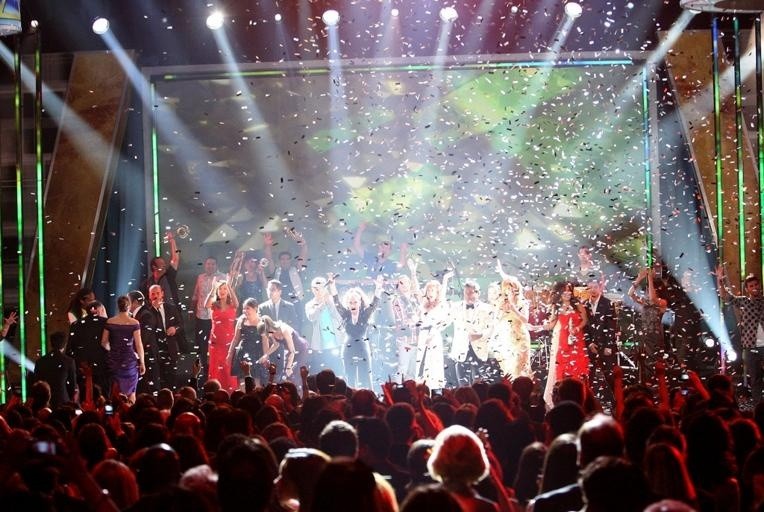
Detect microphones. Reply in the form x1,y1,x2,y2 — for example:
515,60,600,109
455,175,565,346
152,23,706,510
324,272,340,288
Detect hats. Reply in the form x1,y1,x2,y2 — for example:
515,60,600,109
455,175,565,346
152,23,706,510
309,276,326,288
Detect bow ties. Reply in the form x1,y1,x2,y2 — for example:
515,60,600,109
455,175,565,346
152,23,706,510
465,303,475,309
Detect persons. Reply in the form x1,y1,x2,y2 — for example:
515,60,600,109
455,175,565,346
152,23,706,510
578,276,619,399
627,267,668,374
493,258,550,345
0,358,764,512
146,284,184,388
66,302,110,400
0,310,19,342
256,314,309,389
406,258,454,395
256,279,297,334
100,296,146,404
522,281,589,409
713,263,764,407
231,251,268,317
484,281,502,305
263,228,308,340
352,223,408,280
125,290,161,392
32,330,79,407
203,272,239,394
492,277,532,380
195,260,228,379
304,277,346,376
325,272,385,392
226,297,268,388
391,275,421,383
450,281,497,388
67,288,107,324
573,245,605,293
140,231,191,354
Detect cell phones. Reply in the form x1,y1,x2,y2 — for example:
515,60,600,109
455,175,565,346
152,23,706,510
104,401,114,415
28,441,55,456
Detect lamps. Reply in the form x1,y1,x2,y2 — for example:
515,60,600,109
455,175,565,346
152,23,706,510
90,14,109,36
321,9,340,27
563,0,582,18
204,14,223,30
439,7,458,25
